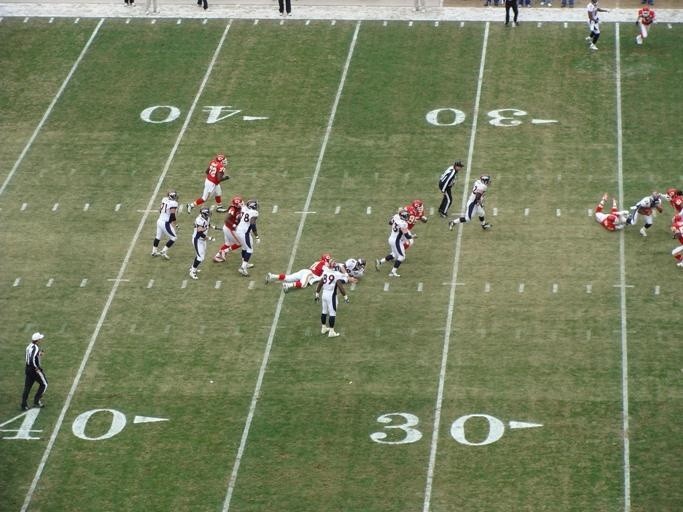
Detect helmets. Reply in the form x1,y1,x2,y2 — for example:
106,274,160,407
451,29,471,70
399,200,423,221
481,176,490,183
232,197,257,209
607,215,618,225
168,193,177,200
321,253,336,268
217,155,227,165
200,208,209,218
651,188,677,203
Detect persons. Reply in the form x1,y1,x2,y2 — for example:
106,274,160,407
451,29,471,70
150,191,178,260
197,0,208,9
438,160,465,218
447,175,494,232
124,0,136,7
189,207,222,280
279,1,292,16
593,186,683,269
21,331,48,411
235,199,261,277
212,197,244,263
186,154,230,215
484,0,656,52
264,199,428,338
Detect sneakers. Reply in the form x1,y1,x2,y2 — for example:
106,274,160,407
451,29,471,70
213,250,226,262
321,327,339,337
603,193,617,207
639,228,647,237
152,250,170,260
238,264,253,275
189,269,201,279
187,204,192,214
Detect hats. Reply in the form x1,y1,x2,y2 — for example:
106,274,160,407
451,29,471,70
455,162,464,166
32,333,43,340
